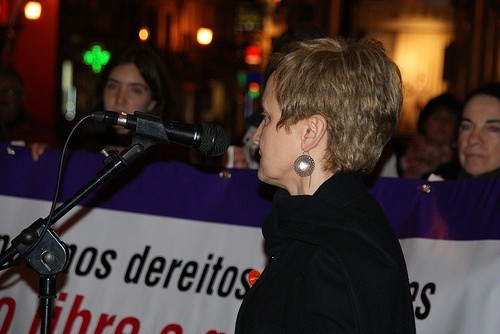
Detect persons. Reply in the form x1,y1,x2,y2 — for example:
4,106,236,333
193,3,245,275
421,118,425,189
0,42,500,180
234,36,416,334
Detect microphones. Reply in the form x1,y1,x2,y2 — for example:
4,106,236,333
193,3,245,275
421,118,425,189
90,111,230,158
